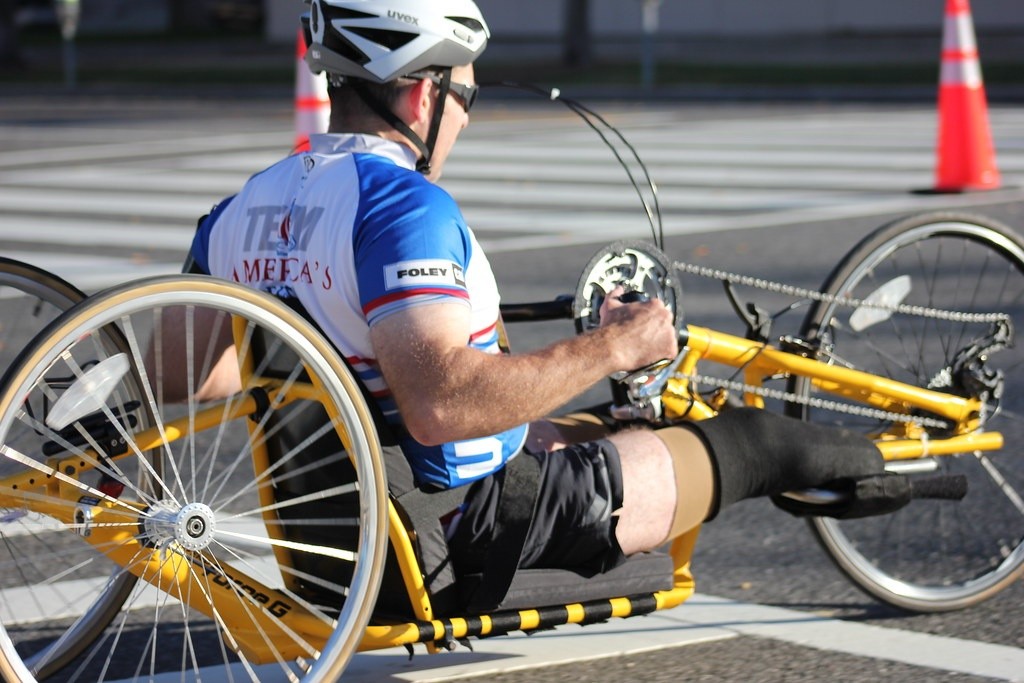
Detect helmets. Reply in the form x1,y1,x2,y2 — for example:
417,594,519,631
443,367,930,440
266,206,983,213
299,0,491,83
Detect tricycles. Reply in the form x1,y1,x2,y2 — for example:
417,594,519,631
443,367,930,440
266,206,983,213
0,74,1023,683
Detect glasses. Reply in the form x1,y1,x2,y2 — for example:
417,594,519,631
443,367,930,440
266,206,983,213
403,73,480,113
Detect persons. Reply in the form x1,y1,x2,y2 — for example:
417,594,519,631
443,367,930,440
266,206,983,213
144,0,885,576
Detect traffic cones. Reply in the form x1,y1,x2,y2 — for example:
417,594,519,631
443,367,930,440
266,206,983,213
911,1,1019,198
288,22,336,160
